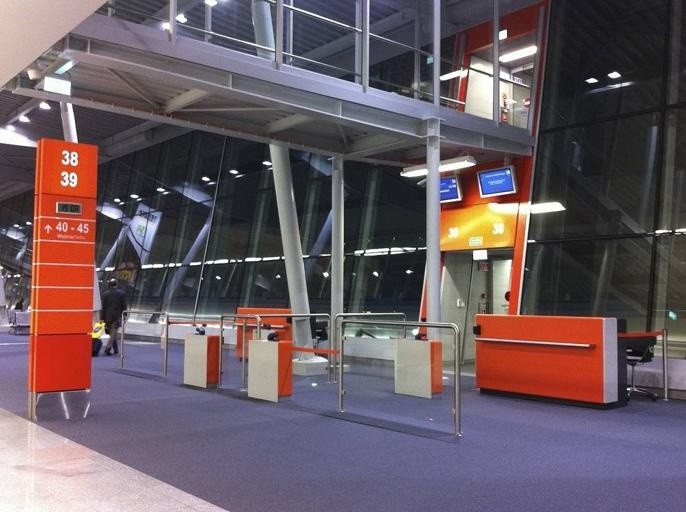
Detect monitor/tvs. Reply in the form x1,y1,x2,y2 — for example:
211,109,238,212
476,165,518,199
440,174,463,203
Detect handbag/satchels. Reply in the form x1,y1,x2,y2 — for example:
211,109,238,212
90,322,106,339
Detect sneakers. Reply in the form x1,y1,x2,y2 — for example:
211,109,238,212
105,350,118,355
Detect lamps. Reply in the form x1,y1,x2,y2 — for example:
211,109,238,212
400,151,477,179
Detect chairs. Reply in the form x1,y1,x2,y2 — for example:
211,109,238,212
6,309,30,335
624,333,658,401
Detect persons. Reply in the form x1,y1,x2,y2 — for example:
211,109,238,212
97,278,127,355
14,297,24,310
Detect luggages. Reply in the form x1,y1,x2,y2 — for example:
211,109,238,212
92,338,102,357
